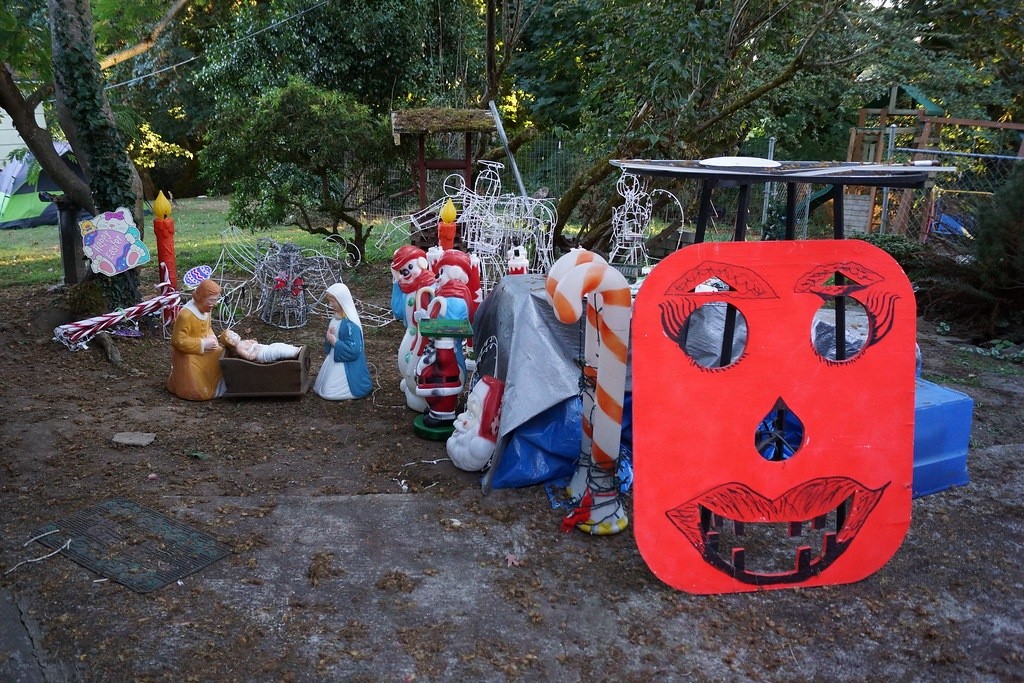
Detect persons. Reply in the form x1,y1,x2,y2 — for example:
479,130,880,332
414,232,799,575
221,329,303,364
167,279,227,400
312,283,372,400
